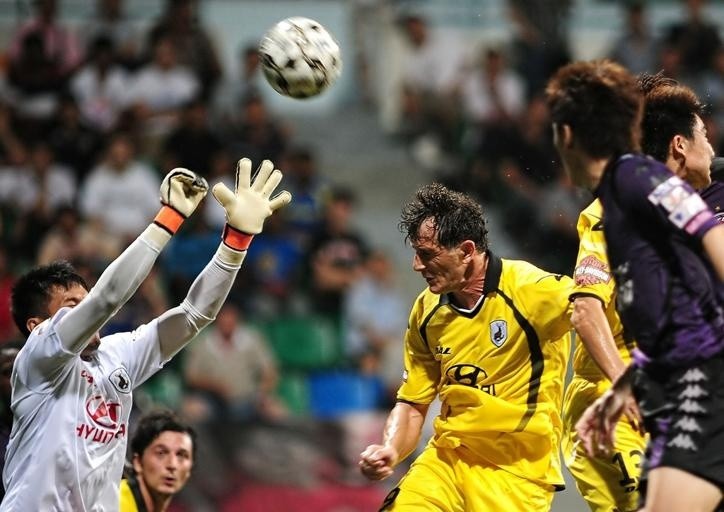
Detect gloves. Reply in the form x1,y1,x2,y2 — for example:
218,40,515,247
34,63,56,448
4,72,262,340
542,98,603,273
153,167,210,236
211,157,293,251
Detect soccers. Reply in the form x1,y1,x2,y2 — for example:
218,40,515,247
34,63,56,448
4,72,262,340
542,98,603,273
258,17,343,98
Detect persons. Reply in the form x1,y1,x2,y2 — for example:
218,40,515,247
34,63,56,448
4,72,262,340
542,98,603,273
1,1,723,512
